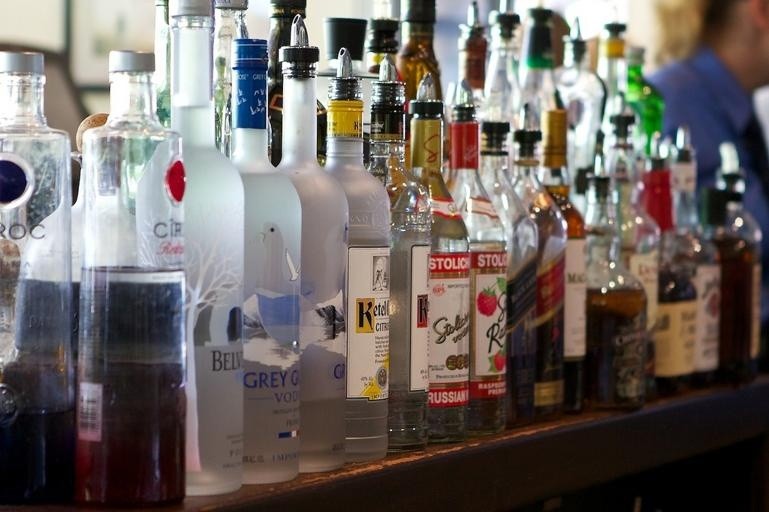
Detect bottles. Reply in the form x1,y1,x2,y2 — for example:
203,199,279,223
442,105,508,438
265,0,327,169
276,14,348,473
672,148,722,385
326,17,372,170
406,102,471,445
538,110,583,414
562,18,606,217
222,41,302,483
524,8,565,127
457,1,486,108
583,176,651,412
718,170,762,382
169,1,244,494
516,129,568,416
325,49,392,465
0,53,73,511
486,0,530,127
152,2,170,132
603,23,632,168
640,156,696,392
481,123,538,430
368,0,399,86
369,80,433,454
611,117,659,402
211,1,251,160
399,1,444,106
77,51,183,511
624,47,663,154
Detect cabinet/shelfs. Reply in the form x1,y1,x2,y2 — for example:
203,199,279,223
0,373,769,512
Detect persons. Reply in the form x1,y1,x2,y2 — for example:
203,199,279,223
647,0,769,324
